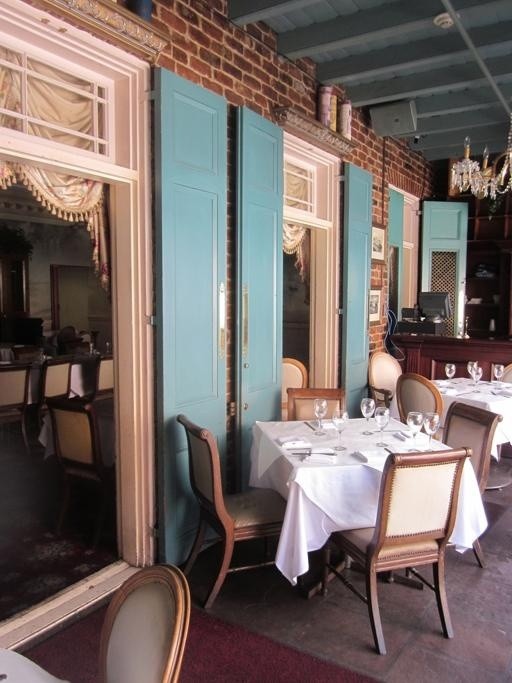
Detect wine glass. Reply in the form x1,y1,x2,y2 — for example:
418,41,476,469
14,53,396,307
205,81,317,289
331,408,350,452
423,413,442,453
359,398,375,436
470,366,483,394
405,411,424,453
374,407,392,448
445,364,457,389
493,364,505,390
466,361,478,386
312,398,328,437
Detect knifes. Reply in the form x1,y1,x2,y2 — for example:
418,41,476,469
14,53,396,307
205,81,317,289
292,451,337,456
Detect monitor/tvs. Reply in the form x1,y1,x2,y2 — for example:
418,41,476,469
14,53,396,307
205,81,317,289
418,290,453,321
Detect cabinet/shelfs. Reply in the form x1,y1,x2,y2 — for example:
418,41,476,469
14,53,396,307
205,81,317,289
460,196,512,337
385,333,512,380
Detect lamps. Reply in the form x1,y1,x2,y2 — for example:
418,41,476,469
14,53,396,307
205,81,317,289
450,104,511,198
433,12,457,30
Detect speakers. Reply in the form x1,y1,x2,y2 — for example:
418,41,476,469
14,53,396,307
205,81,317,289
369,99,418,137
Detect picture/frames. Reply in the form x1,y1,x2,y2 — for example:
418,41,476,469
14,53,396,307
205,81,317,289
50,262,112,351
368,285,383,325
371,220,389,266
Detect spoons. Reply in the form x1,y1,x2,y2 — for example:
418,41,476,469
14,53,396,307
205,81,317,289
490,390,501,395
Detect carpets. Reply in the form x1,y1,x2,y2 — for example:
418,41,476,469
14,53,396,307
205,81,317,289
22,596,388,682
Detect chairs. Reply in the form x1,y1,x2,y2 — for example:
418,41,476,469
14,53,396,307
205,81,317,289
321,446,474,654
397,372,444,440
44,401,116,548
11,346,46,358
368,350,403,407
388,400,505,578
177,414,305,608
0,363,33,451
497,363,512,382
286,387,347,420
73,356,113,412
281,357,309,421
17,357,72,444
51,325,91,352
99,562,192,682
